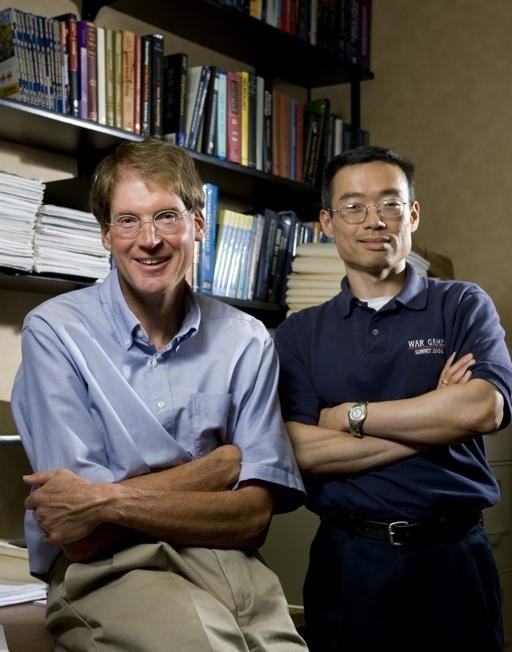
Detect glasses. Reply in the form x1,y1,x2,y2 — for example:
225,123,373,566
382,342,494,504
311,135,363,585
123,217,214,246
326,199,408,222
105,207,191,241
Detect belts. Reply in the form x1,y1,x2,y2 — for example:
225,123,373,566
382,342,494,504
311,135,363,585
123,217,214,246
336,513,482,545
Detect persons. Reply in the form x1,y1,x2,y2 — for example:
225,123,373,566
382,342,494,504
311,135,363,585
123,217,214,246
9,142,314,652
265,146,511,651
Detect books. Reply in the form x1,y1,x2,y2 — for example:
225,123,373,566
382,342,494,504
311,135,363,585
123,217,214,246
1,7,352,184
359,130,370,147
230,1,368,66
0,536,48,609
1,171,430,321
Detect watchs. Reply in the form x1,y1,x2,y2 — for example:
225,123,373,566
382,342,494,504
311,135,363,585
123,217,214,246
346,393,372,439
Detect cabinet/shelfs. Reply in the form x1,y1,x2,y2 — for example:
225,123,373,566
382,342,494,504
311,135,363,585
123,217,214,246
0,0,374,513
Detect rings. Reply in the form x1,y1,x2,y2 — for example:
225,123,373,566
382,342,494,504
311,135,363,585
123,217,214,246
440,378,449,384
